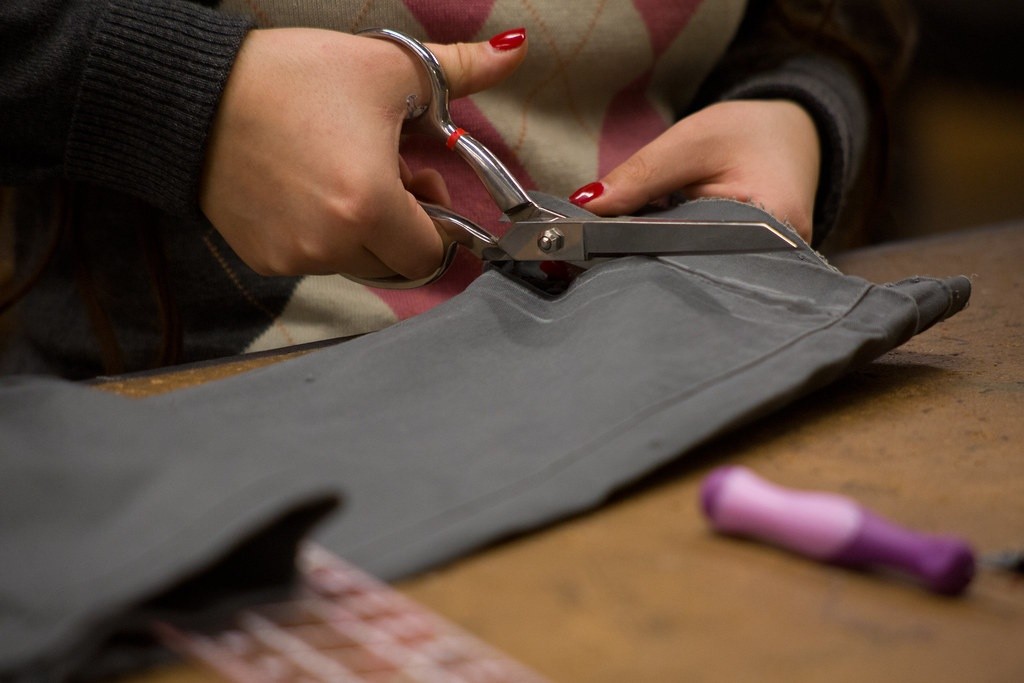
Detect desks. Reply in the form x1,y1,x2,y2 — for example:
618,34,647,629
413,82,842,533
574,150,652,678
0,225,1023,682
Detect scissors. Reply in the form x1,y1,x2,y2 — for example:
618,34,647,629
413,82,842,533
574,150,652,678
336,28,801,292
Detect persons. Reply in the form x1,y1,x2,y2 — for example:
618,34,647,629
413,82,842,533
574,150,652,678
1,1,935,389
